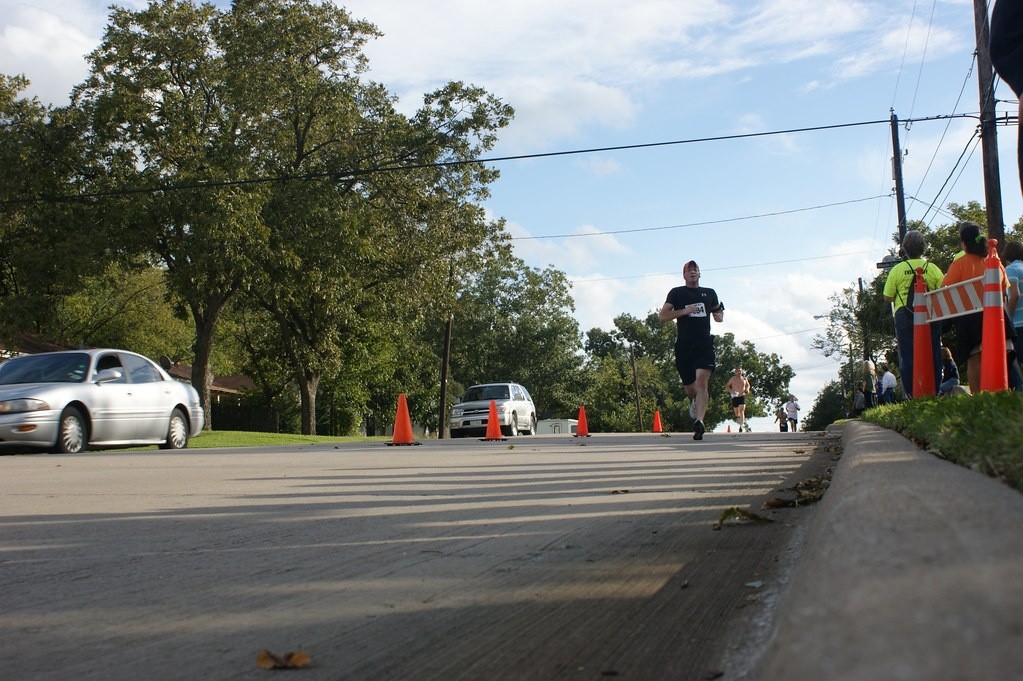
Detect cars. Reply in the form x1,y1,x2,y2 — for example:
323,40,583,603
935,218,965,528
0,348,205,455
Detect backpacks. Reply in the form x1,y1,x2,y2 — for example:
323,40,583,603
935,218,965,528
896,260,929,318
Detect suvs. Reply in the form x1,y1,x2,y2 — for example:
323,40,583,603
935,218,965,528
449,382,538,438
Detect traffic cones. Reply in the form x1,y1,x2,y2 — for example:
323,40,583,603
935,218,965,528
979,239,1008,391
478,400,509,442
651,411,663,432
912,266,936,397
572,405,592,437
727,424,731,432
384,393,422,446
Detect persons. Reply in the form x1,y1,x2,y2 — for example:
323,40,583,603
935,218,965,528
882,229,945,401
726,367,750,433
852,360,898,418
659,260,725,440
785,395,800,433
938,221,1023,395
774,408,788,432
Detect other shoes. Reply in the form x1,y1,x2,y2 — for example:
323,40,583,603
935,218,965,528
689,397,697,419
693,419,705,440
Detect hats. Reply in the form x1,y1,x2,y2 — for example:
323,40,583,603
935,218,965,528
683,260,699,269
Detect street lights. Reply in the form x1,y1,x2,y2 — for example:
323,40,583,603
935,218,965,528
813,315,870,360
